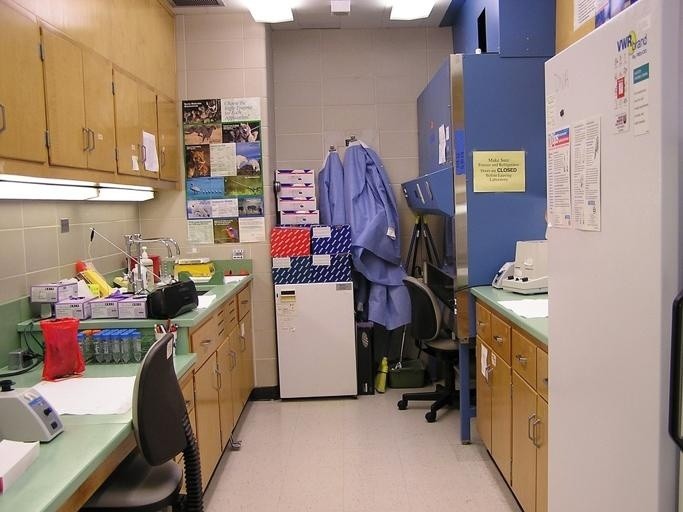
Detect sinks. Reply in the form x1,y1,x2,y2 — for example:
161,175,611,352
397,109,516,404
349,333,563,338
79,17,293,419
195,277,211,298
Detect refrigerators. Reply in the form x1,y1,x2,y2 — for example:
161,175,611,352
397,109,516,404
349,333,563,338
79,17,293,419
544,0,681,512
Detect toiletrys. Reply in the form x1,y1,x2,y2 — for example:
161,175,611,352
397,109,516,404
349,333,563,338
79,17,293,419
141,247,154,289
133,262,148,292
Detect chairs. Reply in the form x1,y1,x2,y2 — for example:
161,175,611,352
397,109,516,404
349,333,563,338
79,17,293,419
396,276,477,422
78,332,205,512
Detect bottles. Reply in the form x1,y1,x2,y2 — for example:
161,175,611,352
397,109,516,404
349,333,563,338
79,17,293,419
74,328,140,364
133,263,148,289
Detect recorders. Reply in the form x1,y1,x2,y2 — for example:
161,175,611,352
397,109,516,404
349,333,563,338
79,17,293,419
145,280,200,320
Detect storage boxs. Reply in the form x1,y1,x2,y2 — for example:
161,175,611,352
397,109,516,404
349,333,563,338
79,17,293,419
268,166,354,289
389,357,425,390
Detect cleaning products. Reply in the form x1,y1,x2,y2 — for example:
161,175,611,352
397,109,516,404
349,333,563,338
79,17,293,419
374,356,387,393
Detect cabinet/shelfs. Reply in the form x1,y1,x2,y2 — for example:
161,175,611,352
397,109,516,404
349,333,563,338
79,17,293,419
158,94,181,182
510,329,548,511
18,282,257,501
41,21,115,174
0,0,46,167
473,299,513,493
113,63,157,181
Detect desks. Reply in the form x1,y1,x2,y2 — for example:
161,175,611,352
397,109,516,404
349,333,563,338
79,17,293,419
1,351,197,511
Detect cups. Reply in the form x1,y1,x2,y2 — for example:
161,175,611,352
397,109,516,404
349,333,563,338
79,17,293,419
154,331,177,358
38,318,85,381
158,258,175,282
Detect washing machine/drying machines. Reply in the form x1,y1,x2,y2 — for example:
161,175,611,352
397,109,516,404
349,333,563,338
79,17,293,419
275,282,359,400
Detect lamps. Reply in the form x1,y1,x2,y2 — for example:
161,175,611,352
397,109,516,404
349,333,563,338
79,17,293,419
0,177,155,203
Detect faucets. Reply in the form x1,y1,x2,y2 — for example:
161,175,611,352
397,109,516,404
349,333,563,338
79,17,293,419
141,237,181,257
133,239,172,259
